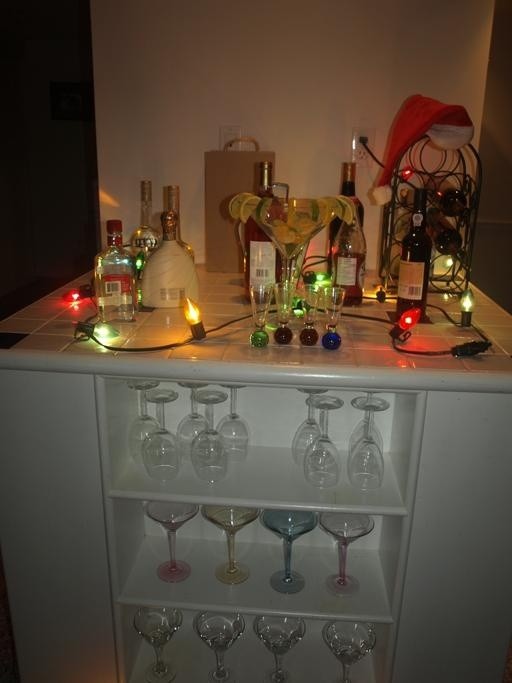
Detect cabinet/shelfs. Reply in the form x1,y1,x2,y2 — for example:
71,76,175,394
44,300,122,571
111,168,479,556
0,370,510,679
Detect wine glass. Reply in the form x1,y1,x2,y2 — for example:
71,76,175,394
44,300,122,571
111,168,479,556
290,387,393,496
143,501,199,583
319,620,377,683
253,614,308,683
192,610,247,682
200,504,261,586
258,509,317,595
125,379,251,490
132,605,184,683
247,281,347,350
319,511,376,599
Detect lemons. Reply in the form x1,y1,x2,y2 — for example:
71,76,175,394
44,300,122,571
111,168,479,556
227,192,356,258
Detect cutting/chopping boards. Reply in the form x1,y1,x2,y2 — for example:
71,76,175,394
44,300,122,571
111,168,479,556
204,136,278,274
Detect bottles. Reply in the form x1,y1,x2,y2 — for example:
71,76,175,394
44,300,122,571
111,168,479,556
395,186,432,323
89,178,199,323
243,160,282,304
326,160,367,307
